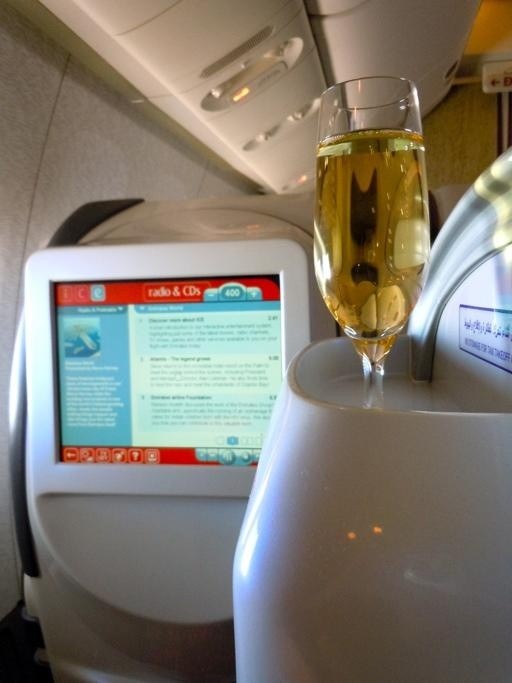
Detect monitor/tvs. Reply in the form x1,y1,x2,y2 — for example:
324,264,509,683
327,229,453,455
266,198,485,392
22,239,310,497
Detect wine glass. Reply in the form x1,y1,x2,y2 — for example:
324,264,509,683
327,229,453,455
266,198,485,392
311,72,434,400
